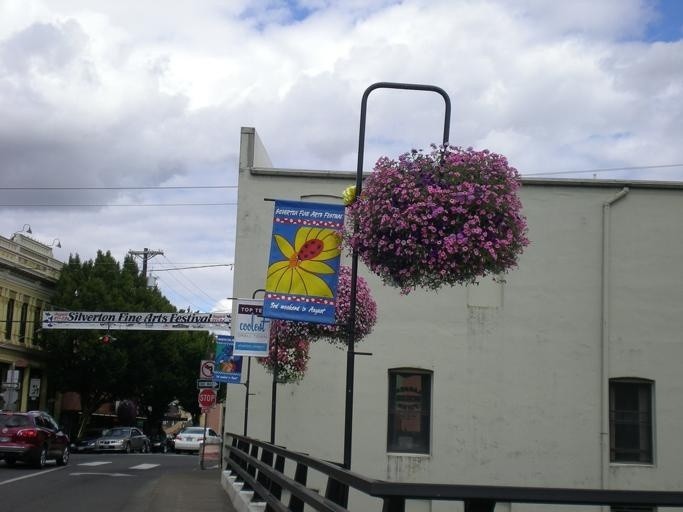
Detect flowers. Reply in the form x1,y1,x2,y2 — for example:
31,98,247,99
253,318,312,388
331,140,530,298
278,264,378,347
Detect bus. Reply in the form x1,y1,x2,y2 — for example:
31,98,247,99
155,411,192,443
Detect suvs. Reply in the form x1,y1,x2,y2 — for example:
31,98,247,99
0,409,70,469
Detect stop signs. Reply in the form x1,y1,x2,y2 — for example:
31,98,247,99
199,391,217,409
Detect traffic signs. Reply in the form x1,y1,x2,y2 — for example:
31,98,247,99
198,380,220,389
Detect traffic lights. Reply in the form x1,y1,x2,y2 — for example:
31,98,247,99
99,335,115,343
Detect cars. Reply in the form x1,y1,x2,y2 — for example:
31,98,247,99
174,427,217,454
72,427,167,454
